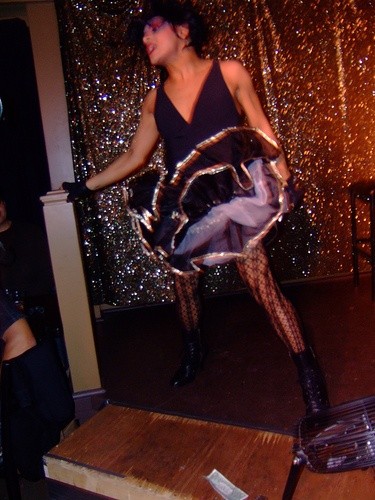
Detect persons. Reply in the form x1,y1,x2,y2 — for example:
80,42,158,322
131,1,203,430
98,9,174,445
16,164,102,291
0,188,76,500
61,0,334,431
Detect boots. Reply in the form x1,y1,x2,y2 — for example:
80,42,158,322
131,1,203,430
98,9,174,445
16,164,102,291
293,347,331,436
171,329,212,386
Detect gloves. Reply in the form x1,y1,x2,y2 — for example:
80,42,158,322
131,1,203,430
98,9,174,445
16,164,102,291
61,180,94,202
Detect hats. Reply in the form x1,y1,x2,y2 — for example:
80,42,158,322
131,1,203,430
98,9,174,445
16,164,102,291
127,0,209,50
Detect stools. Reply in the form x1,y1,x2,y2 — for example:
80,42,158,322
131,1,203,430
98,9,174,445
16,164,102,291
350,179,375,287
